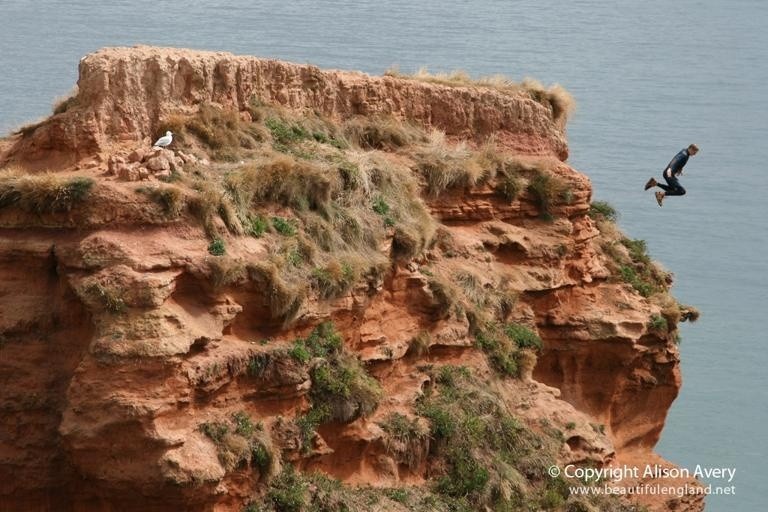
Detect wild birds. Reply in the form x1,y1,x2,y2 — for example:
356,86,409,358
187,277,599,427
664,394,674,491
154,130,175,148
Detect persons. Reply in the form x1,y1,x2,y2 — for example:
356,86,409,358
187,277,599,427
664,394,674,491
644,143,698,207
